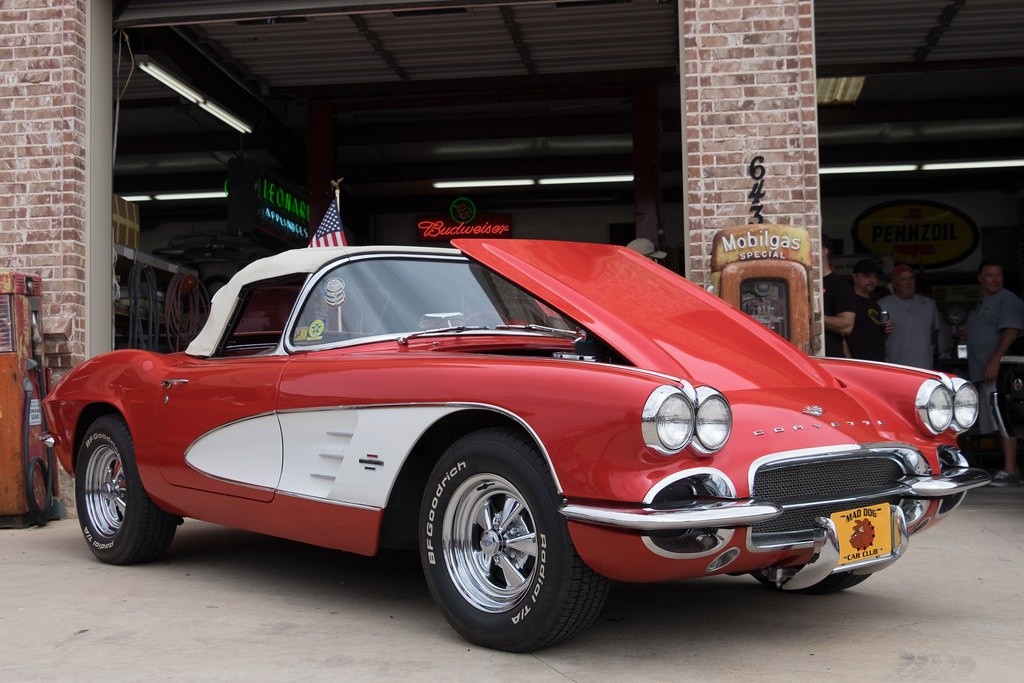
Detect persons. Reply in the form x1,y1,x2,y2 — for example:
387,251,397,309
627,238,656,262
957,262,1024,487
817,235,939,370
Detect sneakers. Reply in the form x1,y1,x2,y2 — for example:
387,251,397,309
987,470,1024,487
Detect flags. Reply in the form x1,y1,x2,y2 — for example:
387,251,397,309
308,199,347,246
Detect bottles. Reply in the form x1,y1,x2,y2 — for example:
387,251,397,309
958,330,966,345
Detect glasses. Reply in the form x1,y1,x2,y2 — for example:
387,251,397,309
645,244,658,256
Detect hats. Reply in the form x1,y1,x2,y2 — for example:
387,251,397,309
854,259,885,276
626,239,668,259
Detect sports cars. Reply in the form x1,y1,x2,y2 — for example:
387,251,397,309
36,235,993,657
